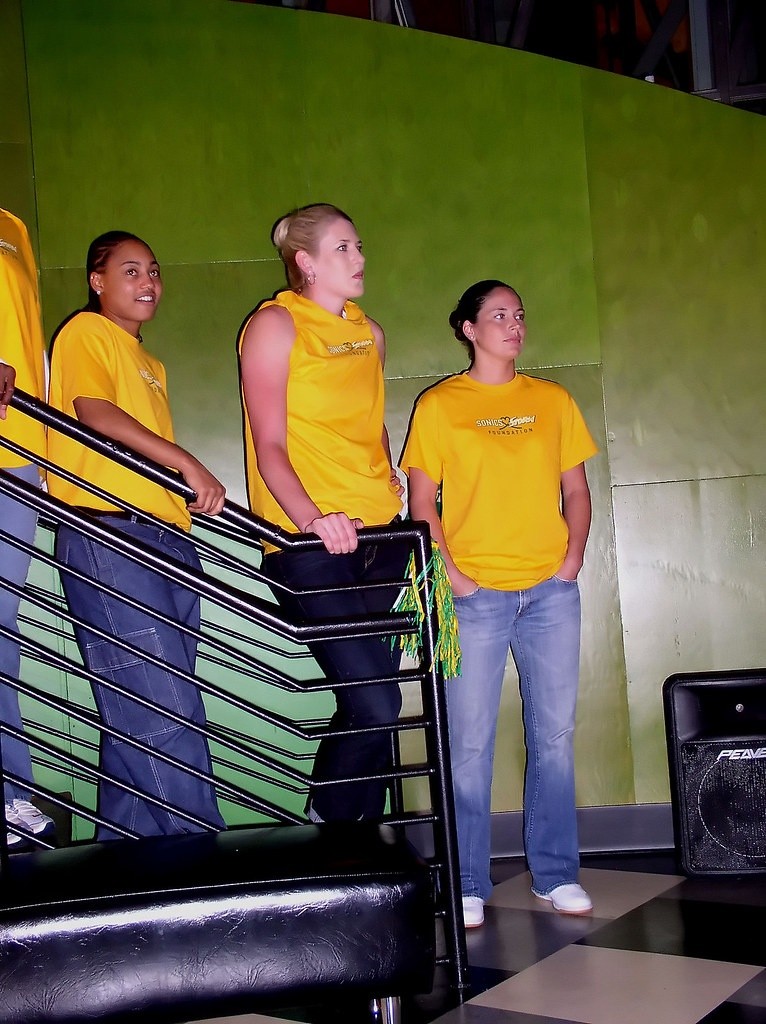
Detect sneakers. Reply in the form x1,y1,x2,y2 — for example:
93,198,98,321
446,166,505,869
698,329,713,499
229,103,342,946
5,805,35,848
533,884,593,915
463,894,485,928
13,799,55,837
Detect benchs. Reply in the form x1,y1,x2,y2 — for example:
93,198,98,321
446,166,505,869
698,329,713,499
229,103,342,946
0,821,438,1023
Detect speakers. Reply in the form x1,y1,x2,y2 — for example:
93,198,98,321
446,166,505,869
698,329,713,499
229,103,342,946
661,668,766,884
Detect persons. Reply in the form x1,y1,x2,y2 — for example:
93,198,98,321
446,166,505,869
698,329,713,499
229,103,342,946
46,230,227,841
237,204,409,823
0,207,55,846
399,281,599,928
